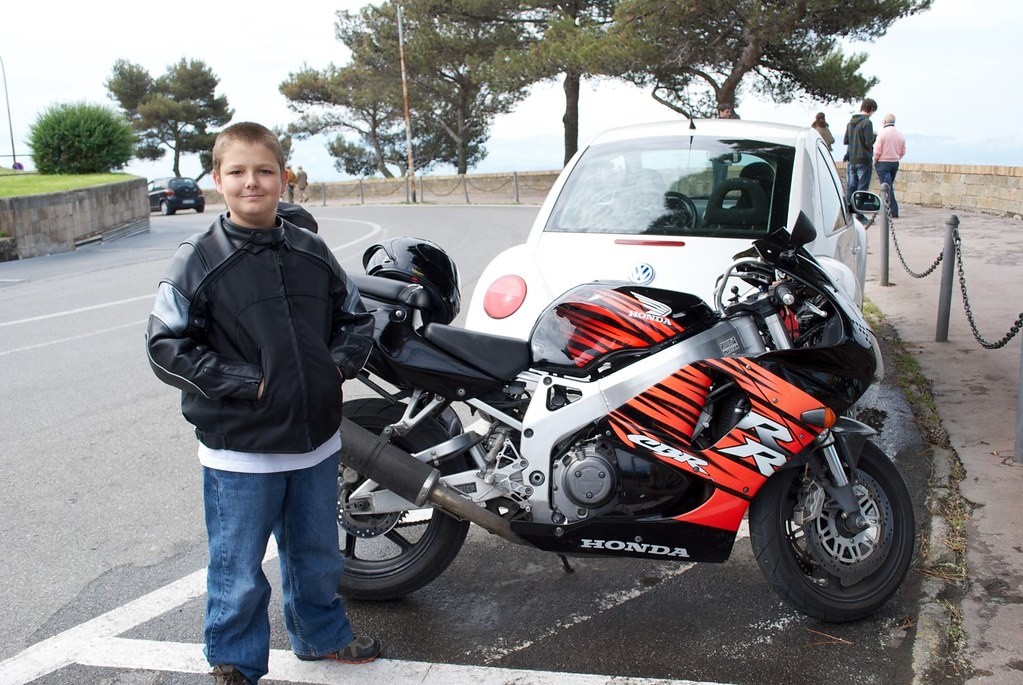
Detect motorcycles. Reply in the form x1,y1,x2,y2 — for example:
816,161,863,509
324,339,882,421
331,236,916,625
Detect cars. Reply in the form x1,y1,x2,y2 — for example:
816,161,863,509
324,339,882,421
461,116,884,355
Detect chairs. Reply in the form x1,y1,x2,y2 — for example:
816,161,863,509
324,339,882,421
701,178,768,230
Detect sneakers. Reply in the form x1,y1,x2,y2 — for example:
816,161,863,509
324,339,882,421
207,663,258,685
294,633,379,664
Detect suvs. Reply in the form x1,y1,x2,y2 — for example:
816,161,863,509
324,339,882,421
148,178,205,216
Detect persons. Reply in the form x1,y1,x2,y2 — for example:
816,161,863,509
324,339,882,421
717,105,731,118
844,98,878,214
812,112,834,153
284,166,310,204
874,114,906,217
145,123,381,684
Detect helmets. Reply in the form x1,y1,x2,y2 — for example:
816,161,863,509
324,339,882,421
362,236,461,326
